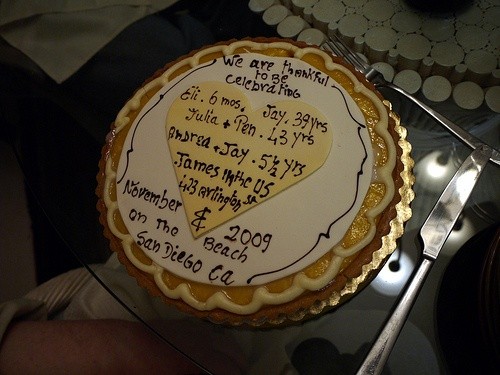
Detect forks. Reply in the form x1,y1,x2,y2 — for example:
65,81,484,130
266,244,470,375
323,33,500,165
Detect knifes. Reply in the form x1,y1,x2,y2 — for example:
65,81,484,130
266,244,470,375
356,144,494,375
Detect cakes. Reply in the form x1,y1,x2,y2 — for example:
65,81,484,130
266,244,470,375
96,39,414,325
